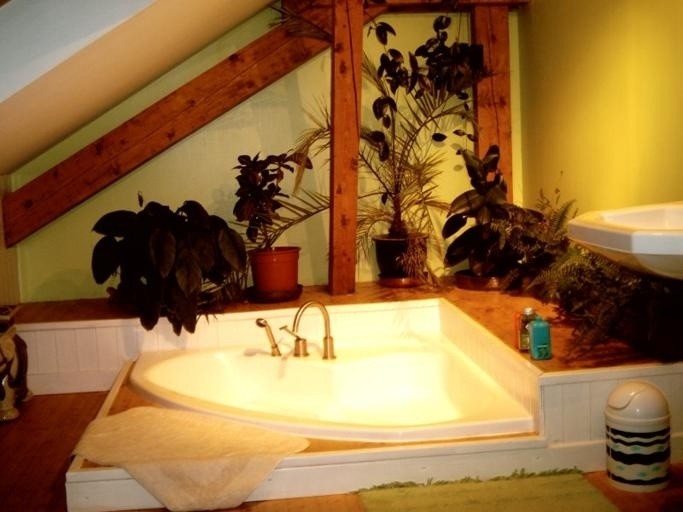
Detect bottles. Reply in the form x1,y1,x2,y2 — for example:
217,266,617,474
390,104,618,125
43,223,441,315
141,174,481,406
527,316,550,360
517,309,538,351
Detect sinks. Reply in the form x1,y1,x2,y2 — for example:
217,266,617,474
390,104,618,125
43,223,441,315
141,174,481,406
130,351,532,436
561,199,682,280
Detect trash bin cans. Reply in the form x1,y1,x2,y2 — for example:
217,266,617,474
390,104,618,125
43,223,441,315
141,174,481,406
603,381,671,495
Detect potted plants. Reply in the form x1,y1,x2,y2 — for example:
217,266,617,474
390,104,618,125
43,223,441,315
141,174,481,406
349,16,494,281
445,134,541,291
231,145,313,300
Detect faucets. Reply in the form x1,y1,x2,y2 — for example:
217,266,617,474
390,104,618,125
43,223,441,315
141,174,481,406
290,299,338,362
256,316,283,361
